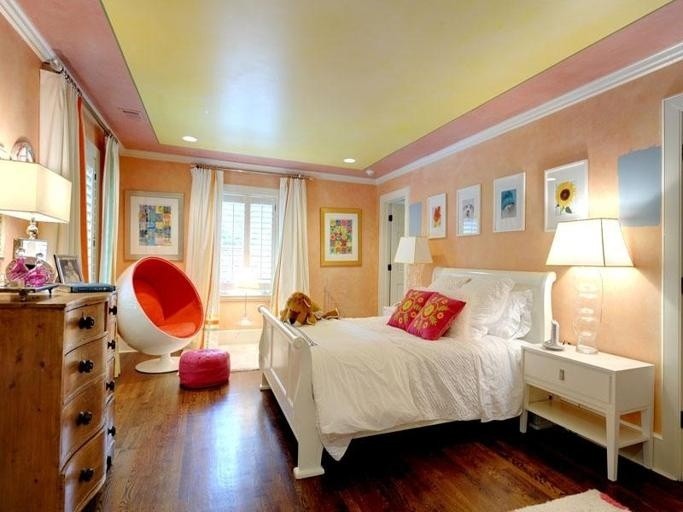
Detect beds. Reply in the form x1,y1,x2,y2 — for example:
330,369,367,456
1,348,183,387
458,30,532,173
257,267,558,481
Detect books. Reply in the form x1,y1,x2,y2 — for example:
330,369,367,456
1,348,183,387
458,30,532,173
70,282,117,293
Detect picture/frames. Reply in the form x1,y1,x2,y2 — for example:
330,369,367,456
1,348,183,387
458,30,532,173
426,192,448,240
492,170,527,234
123,189,185,260
455,183,482,237
319,207,363,267
54,255,84,283
543,158,589,232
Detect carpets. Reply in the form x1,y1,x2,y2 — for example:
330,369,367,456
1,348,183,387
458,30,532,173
508,489,631,511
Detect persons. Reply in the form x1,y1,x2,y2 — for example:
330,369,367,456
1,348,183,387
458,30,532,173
63,262,80,283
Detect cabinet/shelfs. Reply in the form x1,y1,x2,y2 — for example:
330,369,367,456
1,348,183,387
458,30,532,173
1,290,117,512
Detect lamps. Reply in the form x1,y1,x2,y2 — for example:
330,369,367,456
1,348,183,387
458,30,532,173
0,159,73,239
543,219,635,354
394,237,433,288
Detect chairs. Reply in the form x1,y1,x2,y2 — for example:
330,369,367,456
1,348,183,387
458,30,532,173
115,255,204,373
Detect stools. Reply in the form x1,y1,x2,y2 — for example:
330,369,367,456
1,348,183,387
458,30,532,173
178,349,230,390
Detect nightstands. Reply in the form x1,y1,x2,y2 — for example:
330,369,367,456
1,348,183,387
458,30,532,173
519,343,655,481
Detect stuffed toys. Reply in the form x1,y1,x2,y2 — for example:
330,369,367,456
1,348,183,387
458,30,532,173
278,292,320,328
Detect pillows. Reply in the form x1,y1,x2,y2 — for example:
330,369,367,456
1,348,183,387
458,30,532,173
443,279,516,339
488,289,534,339
428,274,472,292
387,289,436,330
406,293,466,339
